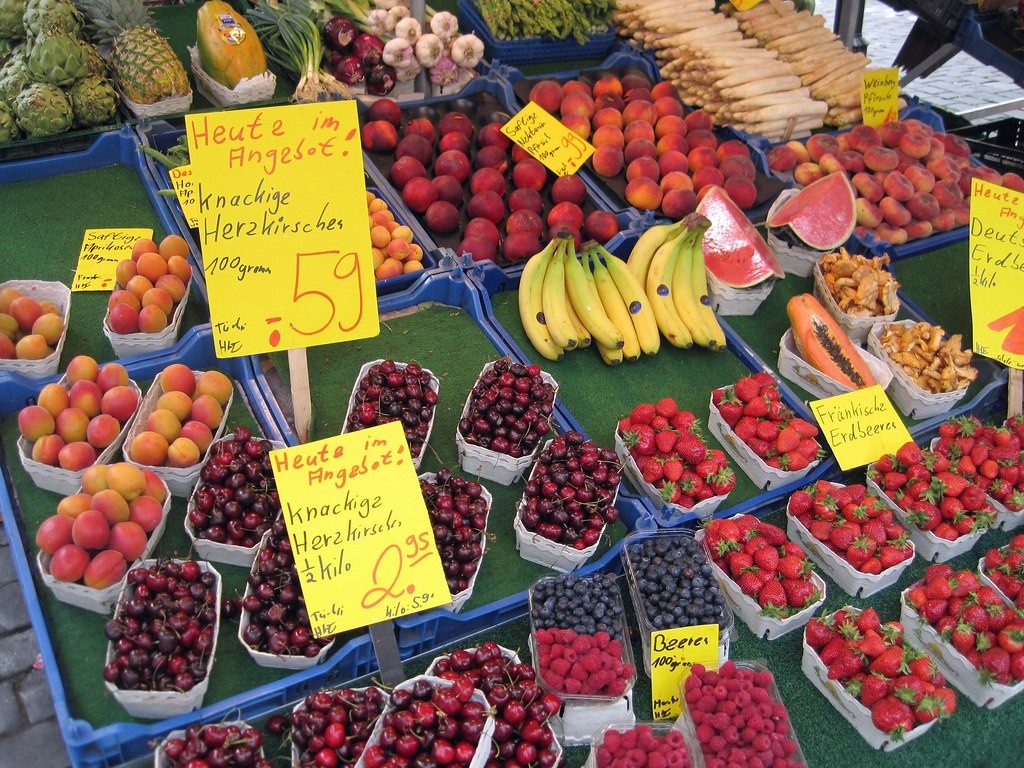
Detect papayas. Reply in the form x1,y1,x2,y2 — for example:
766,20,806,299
785,293,877,391
196,0,267,90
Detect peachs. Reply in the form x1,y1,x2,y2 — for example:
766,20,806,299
531,73,1023,247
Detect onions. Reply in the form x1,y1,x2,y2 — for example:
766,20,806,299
322,17,396,97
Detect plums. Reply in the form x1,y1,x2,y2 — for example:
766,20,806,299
0,234,232,589
366,192,425,280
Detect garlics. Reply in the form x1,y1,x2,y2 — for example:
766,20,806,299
330,0,485,85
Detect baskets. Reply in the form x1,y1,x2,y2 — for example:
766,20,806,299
458,0,616,64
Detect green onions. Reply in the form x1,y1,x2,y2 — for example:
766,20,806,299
241,1,353,103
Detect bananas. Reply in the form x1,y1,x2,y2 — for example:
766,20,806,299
516,214,727,366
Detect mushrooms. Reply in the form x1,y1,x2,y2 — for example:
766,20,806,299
817,246,977,395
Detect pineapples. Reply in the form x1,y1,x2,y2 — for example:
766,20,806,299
76,0,191,105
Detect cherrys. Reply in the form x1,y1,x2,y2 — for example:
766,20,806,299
101,358,621,767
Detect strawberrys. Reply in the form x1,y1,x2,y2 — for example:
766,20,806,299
619,373,1024,742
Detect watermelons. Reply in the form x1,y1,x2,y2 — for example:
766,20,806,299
692,186,784,290
768,171,858,251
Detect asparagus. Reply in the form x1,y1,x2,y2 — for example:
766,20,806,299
475,0,617,46
